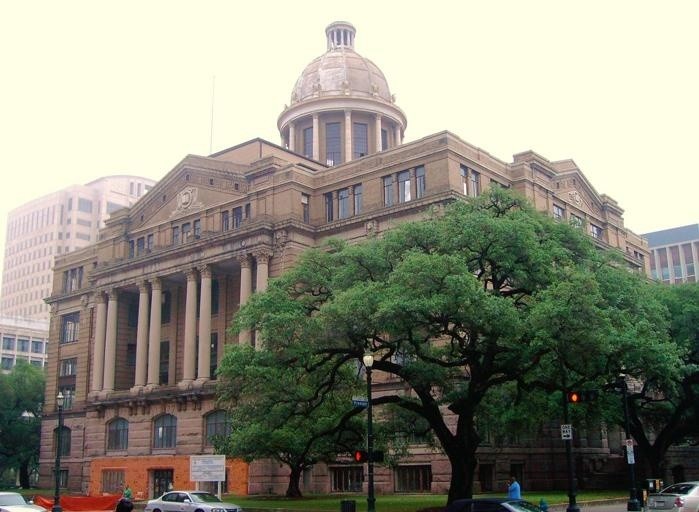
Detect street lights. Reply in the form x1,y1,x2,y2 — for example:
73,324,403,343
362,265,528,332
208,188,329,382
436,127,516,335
51,391,65,512
362,351,376,511
619,361,641,511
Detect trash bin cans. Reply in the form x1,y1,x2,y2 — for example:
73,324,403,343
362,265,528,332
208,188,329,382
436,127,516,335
341,500,356,512
630,489,644,508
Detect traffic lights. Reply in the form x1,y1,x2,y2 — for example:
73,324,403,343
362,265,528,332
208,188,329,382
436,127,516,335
566,390,598,402
355,451,384,463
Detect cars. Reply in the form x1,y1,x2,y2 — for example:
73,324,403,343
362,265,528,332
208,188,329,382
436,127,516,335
0,492,47,512
644,481,699,512
416,498,547,511
145,490,241,512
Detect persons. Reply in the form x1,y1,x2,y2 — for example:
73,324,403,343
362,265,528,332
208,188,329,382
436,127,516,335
115,483,134,512
507,476,522,499
